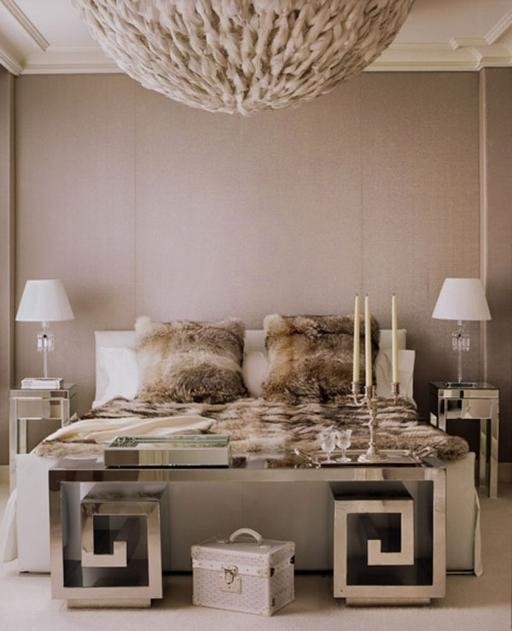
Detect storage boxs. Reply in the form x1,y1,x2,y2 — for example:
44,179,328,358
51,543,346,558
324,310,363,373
191,526,295,617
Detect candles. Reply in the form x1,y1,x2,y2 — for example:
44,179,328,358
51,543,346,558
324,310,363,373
351,294,399,386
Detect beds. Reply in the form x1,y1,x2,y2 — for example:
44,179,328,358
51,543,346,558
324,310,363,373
11,329,482,576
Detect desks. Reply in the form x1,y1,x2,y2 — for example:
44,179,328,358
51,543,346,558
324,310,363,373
44,452,449,610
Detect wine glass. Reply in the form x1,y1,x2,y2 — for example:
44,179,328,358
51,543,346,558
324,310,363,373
335,429,352,461
319,432,337,464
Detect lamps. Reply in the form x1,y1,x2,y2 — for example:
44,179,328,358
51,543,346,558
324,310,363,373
15,279,75,379
431,274,493,386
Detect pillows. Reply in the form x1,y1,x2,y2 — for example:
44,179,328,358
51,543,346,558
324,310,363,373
260,312,383,404
131,312,250,408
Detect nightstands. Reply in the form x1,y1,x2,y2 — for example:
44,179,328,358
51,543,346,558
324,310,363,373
9,384,75,453
430,381,501,501
